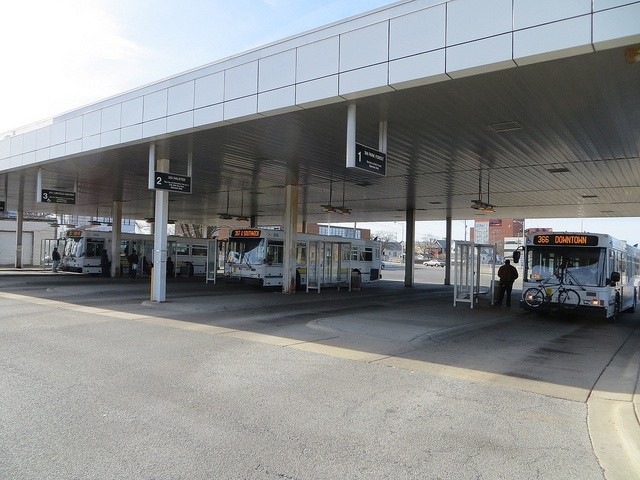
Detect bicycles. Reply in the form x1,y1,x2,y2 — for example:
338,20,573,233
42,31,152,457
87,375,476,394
523,277,581,311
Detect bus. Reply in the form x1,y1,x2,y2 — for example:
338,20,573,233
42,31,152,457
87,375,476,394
222,228,385,292
513,232,640,320
57,228,218,277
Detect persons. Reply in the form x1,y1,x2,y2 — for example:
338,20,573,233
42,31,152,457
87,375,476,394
143,256,148,275
128,250,138,280
166,257,173,277
101,250,111,278
496,260,518,307
52,247,61,273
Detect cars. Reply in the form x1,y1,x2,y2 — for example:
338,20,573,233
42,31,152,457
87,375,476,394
423,259,446,267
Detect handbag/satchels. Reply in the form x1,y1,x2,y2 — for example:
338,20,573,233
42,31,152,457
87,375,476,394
132,264,138,269
